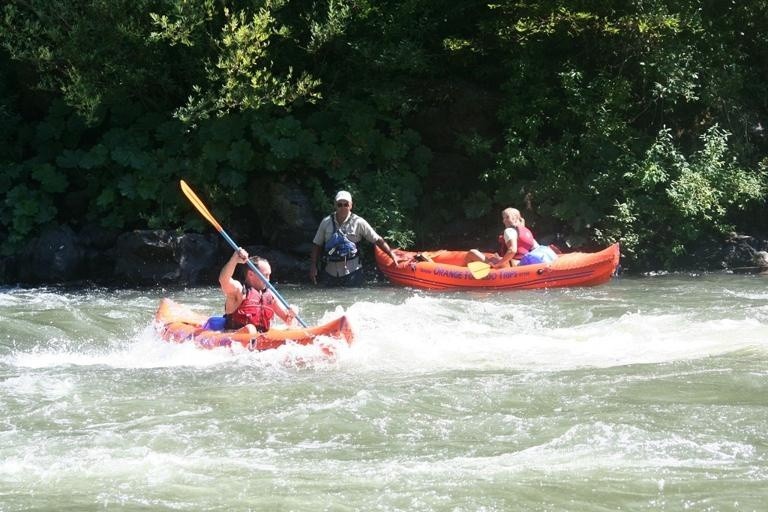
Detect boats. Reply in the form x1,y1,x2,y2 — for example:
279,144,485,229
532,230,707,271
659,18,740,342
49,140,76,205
152,297,353,367
374,241,620,295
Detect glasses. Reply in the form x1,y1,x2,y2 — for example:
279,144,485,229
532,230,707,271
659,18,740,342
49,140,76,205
338,203,350,207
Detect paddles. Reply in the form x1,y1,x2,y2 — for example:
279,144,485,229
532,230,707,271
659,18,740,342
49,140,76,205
467,256,503,280
179,179,309,327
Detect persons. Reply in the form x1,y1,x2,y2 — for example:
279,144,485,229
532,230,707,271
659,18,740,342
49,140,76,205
488,207,540,270
219,246,299,336
310,189,401,290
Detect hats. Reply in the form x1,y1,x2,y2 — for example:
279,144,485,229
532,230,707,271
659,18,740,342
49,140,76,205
335,191,352,202
